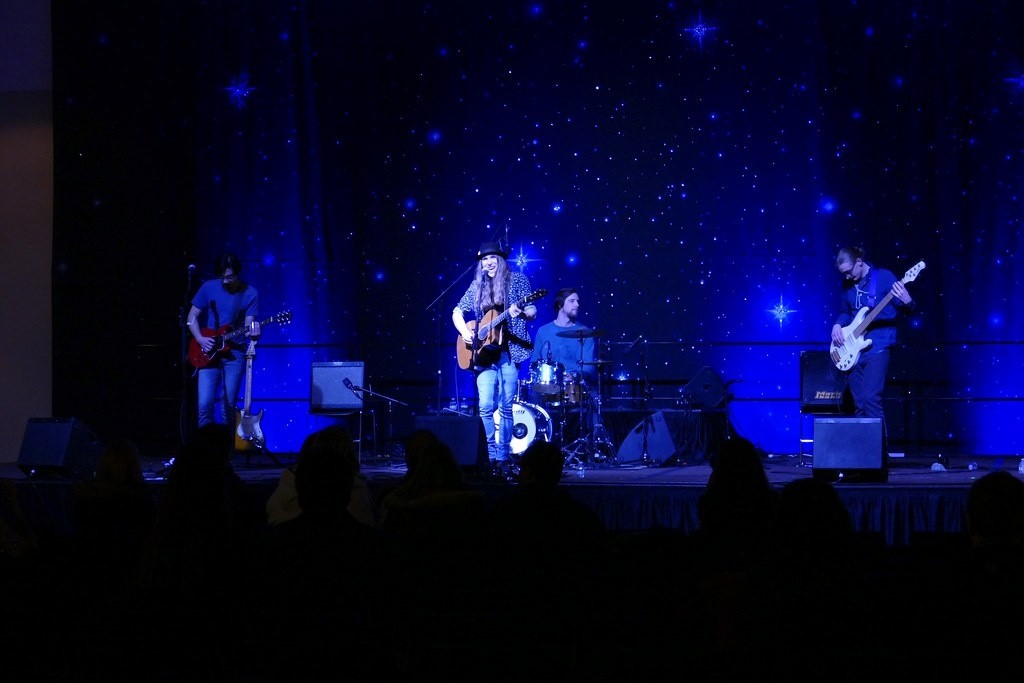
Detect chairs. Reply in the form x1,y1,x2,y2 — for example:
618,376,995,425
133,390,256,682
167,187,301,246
799,350,849,467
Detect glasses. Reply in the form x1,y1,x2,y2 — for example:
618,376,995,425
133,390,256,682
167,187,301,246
845,263,856,274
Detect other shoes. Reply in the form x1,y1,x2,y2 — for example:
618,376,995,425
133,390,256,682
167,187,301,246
491,461,519,476
497,462,515,481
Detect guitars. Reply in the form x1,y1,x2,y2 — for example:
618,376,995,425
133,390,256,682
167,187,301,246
234,337,265,459
188,310,298,368
455,288,549,374
829,260,926,372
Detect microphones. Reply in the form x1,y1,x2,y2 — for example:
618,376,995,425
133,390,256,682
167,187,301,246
343,377,360,398
188,265,195,271
481,267,490,276
547,342,552,360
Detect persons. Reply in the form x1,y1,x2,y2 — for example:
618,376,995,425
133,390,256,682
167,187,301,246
829,245,918,440
530,288,599,443
0,414,1024,683
452,242,538,476
186,252,261,431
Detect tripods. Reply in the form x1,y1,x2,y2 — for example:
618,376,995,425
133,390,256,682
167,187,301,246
560,335,617,468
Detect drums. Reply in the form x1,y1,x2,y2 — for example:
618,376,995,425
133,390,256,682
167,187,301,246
541,370,584,411
493,402,553,457
528,361,562,396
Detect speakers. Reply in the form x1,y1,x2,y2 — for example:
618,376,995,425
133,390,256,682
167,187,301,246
311,362,365,411
16,416,104,481
812,418,887,485
414,416,488,476
617,366,730,465
799,350,854,414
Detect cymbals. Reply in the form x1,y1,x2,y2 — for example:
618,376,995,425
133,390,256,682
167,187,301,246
555,328,610,339
578,360,614,367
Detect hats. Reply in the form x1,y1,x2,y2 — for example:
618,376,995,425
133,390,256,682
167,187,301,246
476,243,507,260
